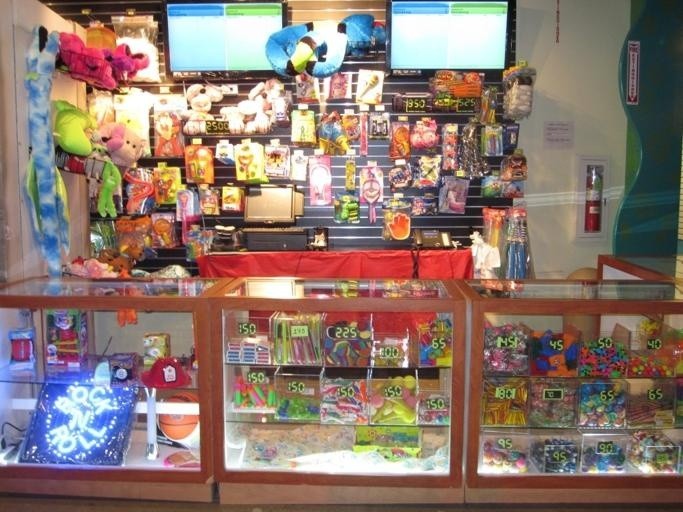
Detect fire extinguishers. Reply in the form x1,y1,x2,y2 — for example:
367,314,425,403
584,166,602,233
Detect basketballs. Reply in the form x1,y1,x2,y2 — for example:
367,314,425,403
157,391,199,440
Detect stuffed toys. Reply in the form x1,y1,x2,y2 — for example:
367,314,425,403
20,20,151,281
264,13,383,77
183,78,289,136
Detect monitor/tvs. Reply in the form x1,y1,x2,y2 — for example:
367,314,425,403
244,182,296,225
384,1,513,80
161,0,288,80
